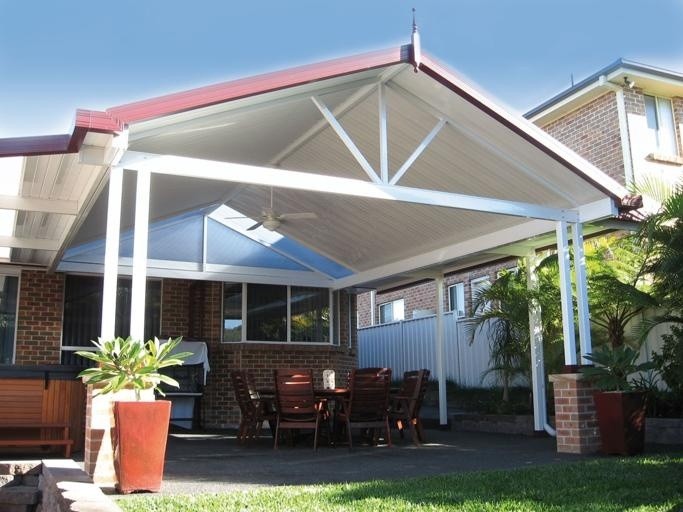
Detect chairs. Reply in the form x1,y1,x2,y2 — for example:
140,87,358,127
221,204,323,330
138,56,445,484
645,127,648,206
230,364,429,454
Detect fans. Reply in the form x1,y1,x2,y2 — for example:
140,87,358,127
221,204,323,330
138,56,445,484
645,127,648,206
221,188,317,232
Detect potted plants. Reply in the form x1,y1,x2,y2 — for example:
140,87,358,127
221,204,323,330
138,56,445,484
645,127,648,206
578,343,651,454
72,333,194,498
